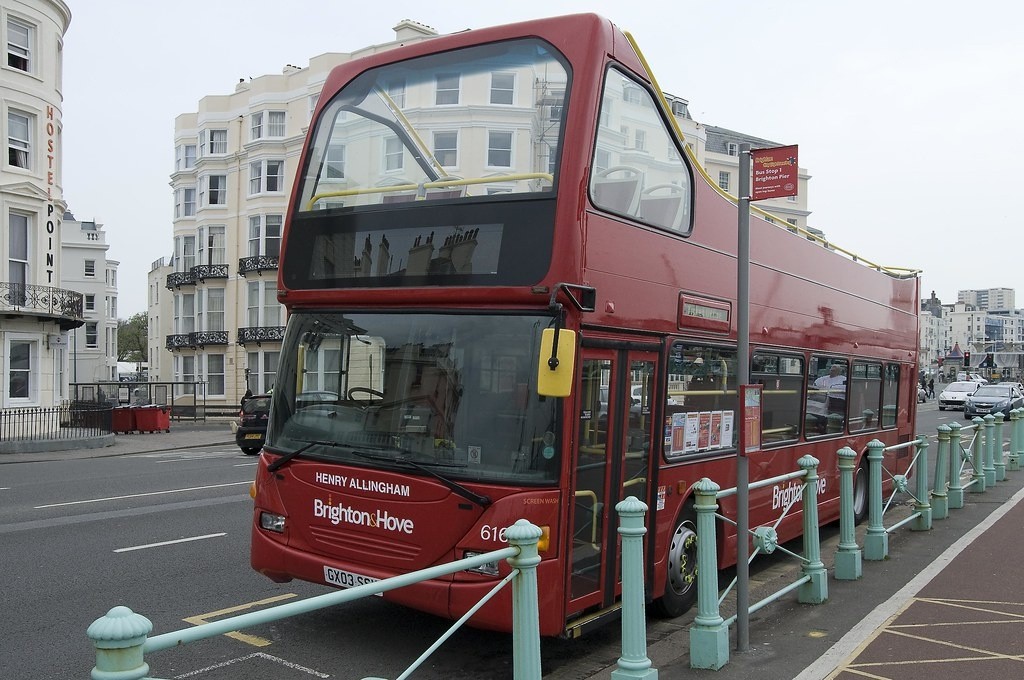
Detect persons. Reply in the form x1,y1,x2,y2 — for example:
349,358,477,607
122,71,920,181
1001,370,1010,382
966,367,970,380
812,364,847,388
942,373,945,379
938,376,940,383
922,376,936,399
987,370,991,383
241,389,252,406
1017,376,1024,388
267,383,274,394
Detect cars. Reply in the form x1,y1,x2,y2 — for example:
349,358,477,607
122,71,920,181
997,382,1024,394
917,387,928,403
939,374,996,411
236,392,344,455
133,385,148,396
964,385,1024,421
599,385,679,423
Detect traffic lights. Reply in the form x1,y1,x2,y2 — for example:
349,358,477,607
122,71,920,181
964,351,970,366
987,354,994,367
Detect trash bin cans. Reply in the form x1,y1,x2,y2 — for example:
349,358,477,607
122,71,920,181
111,406,137,435
134,406,170,435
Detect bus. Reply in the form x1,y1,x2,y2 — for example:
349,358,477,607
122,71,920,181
251,13,923,640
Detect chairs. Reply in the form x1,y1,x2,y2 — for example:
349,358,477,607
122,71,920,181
424,175,467,200
641,183,687,231
378,175,419,203
591,165,645,217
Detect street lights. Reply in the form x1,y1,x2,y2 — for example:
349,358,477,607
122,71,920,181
245,367,251,389
